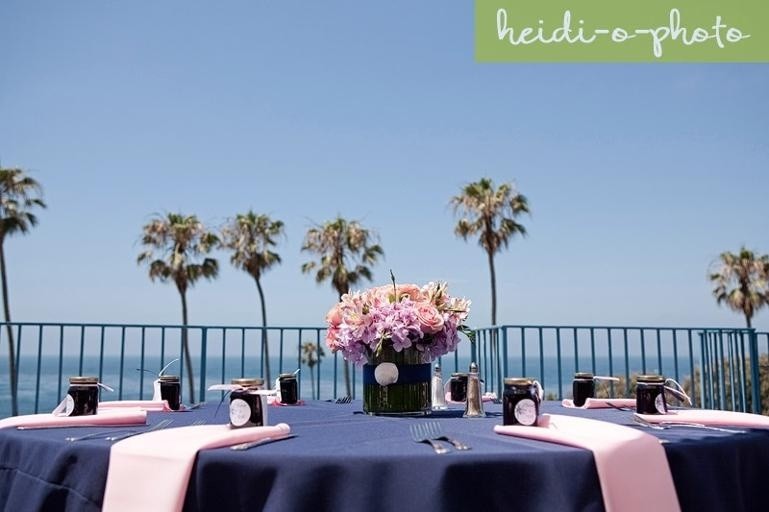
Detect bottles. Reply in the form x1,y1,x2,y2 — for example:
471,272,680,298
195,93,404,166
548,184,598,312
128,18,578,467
433,361,667,426
66,373,300,433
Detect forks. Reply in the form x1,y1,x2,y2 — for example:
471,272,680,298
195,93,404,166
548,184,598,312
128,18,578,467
65,418,208,443
409,420,470,457
323,394,353,404
631,411,748,435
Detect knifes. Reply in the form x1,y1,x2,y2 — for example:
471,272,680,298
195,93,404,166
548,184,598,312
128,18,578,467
231,433,297,449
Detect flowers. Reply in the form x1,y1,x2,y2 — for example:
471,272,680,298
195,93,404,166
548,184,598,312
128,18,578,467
322,281,470,409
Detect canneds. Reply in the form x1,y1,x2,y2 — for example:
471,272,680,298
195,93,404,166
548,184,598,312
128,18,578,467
229,378,267,429
502,378,540,426
636,375,667,415
277,373,297,405
158,374,180,411
67,377,97,416
451,373,468,401
573,373,594,405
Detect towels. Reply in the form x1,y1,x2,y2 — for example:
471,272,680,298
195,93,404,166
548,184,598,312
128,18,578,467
490,395,765,511
0,399,292,511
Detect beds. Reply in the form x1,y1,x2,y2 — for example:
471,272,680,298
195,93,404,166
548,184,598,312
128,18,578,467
0,397,769,511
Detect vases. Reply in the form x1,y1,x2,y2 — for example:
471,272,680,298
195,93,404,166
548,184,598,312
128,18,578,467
361,344,430,416
